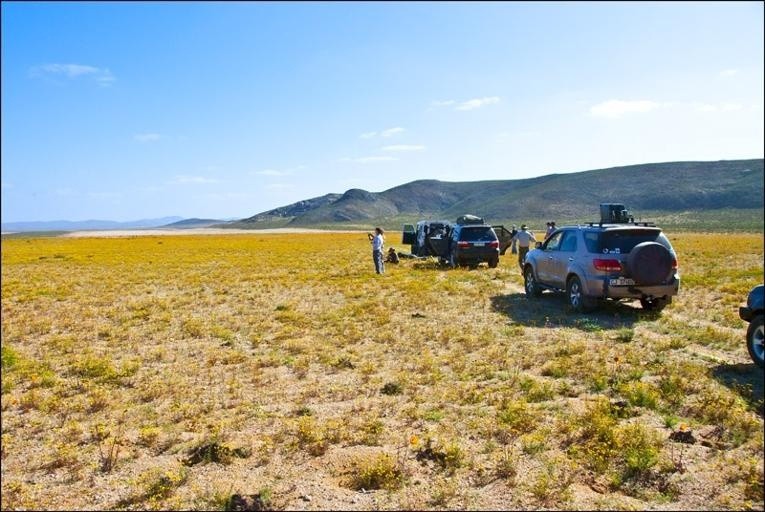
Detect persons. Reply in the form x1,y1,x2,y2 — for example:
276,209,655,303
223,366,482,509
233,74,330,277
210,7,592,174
383,246,400,264
368,227,384,275
510,224,519,254
507,224,537,275
549,221,558,237
544,221,552,240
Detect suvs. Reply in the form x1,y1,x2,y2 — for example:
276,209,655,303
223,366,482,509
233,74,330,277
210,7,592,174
401,221,453,257
424,224,516,270
519,220,680,314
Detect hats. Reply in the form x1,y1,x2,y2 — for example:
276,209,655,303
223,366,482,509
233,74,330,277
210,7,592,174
518,224,529,229
546,222,555,226
